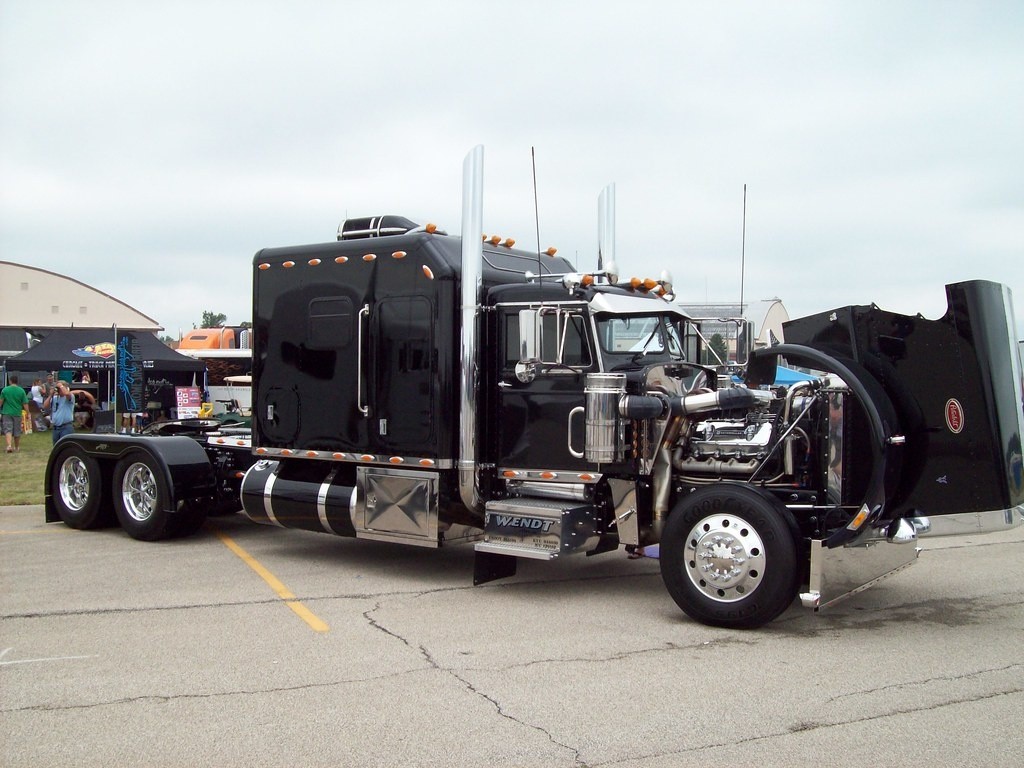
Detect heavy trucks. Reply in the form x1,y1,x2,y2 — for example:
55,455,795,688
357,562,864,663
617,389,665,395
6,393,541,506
44,213,1024,631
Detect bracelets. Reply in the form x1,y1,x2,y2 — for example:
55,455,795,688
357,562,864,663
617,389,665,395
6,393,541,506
58,385,63,389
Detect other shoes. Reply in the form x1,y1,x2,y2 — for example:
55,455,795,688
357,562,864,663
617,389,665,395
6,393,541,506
117,427,126,433
130,428,135,433
6,446,13,453
14,447,20,452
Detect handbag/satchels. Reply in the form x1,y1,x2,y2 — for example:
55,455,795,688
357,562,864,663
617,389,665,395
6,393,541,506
26,392,33,400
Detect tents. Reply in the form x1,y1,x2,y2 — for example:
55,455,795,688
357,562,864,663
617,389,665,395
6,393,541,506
3,330,206,420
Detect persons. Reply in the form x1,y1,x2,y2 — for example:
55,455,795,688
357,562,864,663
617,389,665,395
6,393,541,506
31,378,46,408
82,371,90,384
44,380,75,447
42,374,57,402
0,375,29,453
74,391,91,431
118,413,143,434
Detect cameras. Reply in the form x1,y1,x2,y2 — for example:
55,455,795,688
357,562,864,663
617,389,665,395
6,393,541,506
54,387,59,392
